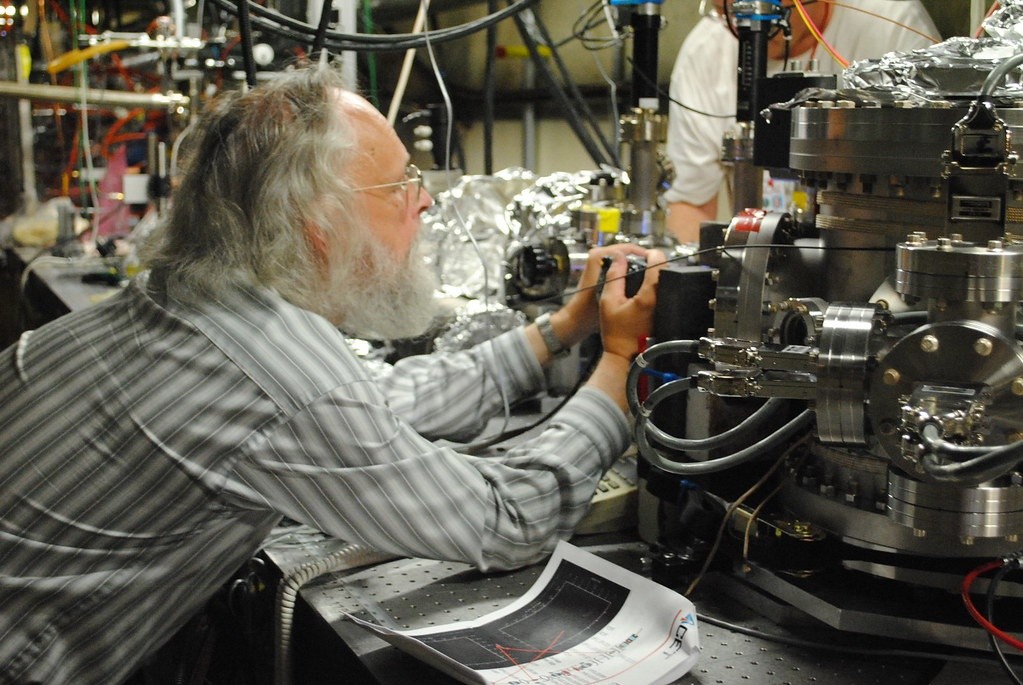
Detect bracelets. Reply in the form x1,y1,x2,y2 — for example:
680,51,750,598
534,312,569,359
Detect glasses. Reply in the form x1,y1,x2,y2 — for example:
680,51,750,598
349,164,423,208
699,0,738,29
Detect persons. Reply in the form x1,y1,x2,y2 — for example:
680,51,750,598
0,79,665,685
664,0,944,245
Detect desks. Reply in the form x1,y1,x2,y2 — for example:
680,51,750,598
10,240,1023,685
